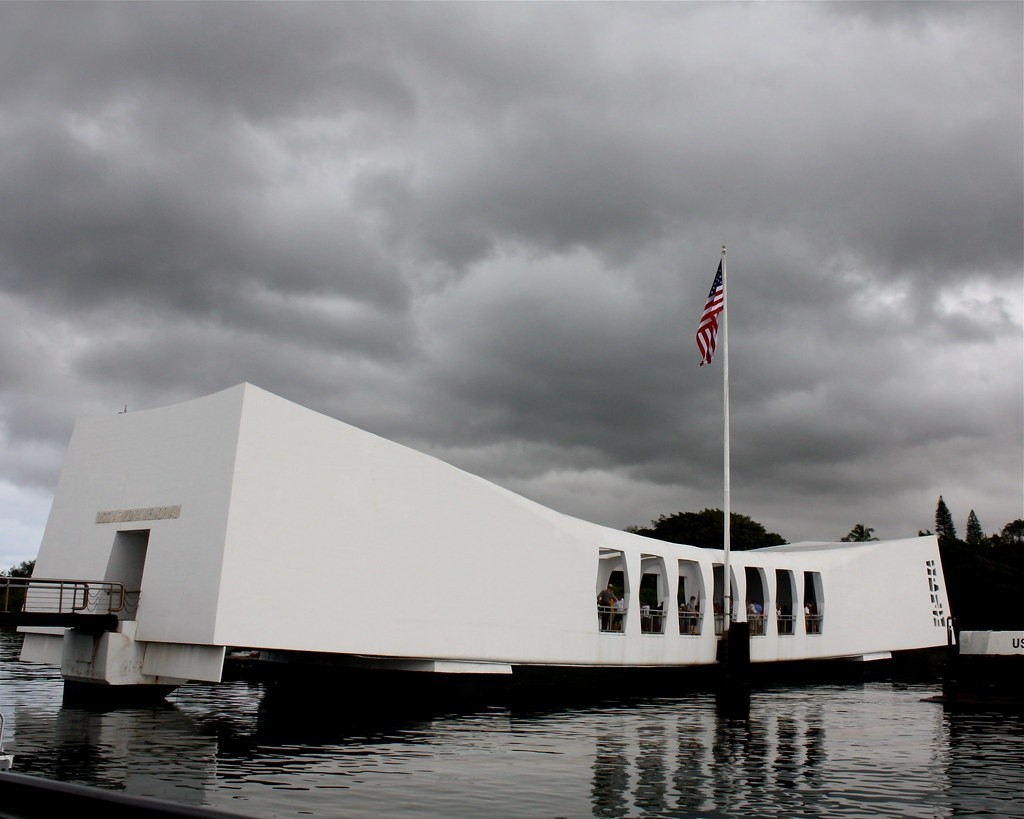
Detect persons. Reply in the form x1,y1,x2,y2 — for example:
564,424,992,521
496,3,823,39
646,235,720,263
805,602,817,632
640,600,663,633
679,596,699,634
713,600,733,618
776,601,792,633
597,584,625,632
747,599,762,634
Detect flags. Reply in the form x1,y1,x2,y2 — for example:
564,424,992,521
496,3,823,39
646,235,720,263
696,259,724,366
